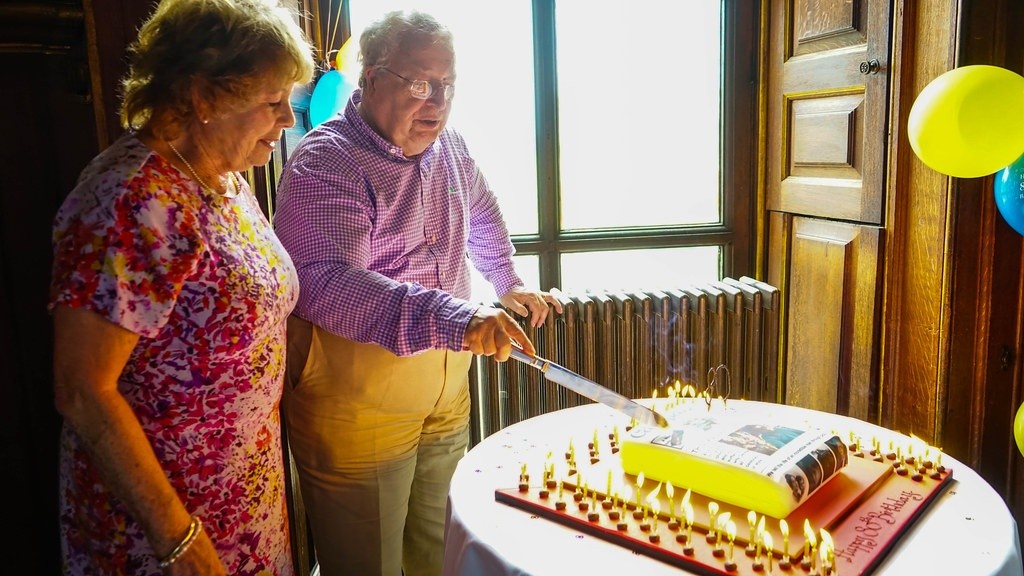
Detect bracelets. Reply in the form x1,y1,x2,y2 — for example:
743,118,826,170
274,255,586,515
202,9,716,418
159,518,196,564
158,513,203,569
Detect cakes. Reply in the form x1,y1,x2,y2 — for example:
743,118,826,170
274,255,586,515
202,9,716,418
620,394,852,522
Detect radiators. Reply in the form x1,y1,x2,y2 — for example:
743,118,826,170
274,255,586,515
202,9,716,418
468,275,780,451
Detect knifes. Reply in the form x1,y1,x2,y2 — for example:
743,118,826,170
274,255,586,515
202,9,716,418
509,342,668,428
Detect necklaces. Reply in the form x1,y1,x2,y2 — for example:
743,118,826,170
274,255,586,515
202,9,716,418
165,138,243,200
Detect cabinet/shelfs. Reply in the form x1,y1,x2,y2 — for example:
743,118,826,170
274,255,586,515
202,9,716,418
766,0,891,224
766,209,886,425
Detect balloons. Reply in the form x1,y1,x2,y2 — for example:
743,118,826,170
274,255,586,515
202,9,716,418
1013,400,1024,457
993,151,1024,236
334,33,367,81
906,63,1024,180
306,69,357,132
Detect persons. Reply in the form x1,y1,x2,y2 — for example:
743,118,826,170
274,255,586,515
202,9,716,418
48,2,301,576
273,9,564,576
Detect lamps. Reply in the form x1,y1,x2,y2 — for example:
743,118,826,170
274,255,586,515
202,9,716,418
519,378,944,576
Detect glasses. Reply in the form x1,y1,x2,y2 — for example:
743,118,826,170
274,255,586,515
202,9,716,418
381,68,454,102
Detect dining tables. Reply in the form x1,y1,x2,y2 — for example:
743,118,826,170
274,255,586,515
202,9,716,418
444,393,1023,575
